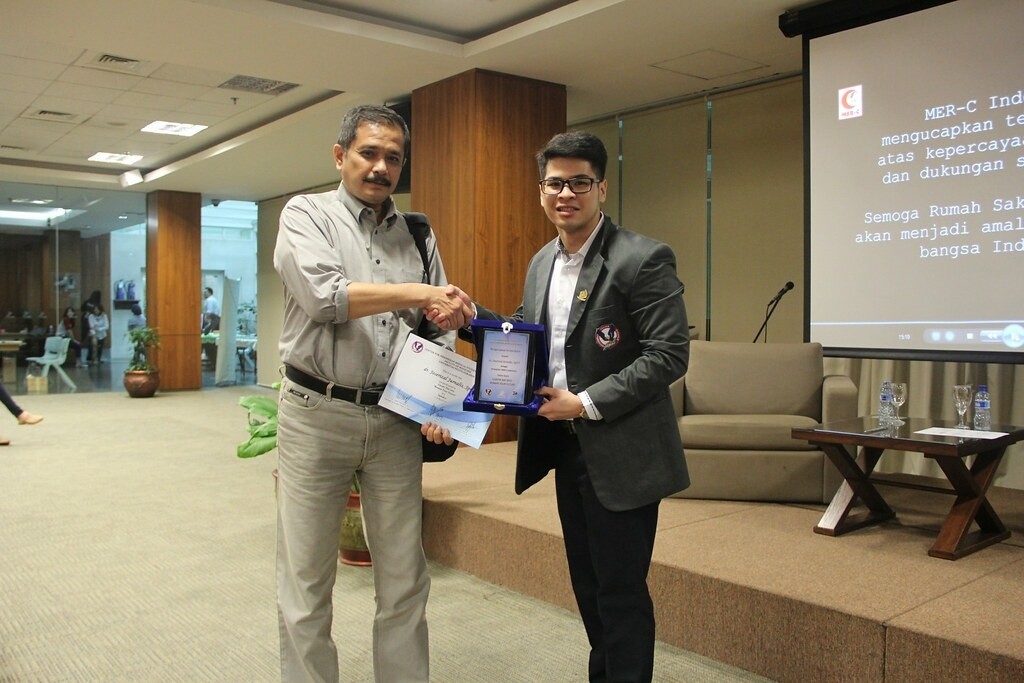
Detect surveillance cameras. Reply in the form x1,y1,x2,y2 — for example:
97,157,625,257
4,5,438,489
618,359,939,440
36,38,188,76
211,199,219,206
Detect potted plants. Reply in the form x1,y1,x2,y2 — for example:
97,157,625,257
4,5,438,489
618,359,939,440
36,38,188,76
237,383,373,566
123,326,163,398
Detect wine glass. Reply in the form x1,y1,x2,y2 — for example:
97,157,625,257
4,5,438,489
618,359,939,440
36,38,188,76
953,386,973,429
890,383,906,426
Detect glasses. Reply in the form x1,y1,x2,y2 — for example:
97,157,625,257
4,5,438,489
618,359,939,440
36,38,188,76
539,177,599,195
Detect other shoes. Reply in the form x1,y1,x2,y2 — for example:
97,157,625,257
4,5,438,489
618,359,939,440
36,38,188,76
18,412,44,426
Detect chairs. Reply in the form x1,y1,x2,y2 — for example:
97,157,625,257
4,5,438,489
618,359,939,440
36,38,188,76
27,336,77,390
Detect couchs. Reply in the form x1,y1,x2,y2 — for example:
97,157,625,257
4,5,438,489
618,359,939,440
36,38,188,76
666,340,858,509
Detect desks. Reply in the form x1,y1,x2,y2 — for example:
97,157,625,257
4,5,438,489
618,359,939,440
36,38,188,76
0,340,27,384
216,338,258,377
791,414,1024,560
200,333,219,371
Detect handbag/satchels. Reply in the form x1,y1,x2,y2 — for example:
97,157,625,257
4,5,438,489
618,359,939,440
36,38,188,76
418,336,460,462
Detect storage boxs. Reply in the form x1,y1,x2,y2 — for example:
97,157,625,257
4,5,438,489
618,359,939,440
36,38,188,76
26,375,48,392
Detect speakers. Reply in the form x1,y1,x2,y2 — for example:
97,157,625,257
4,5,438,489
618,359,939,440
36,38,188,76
119,169,143,189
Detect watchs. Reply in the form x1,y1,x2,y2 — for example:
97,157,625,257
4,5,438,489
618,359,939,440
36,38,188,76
580,407,588,418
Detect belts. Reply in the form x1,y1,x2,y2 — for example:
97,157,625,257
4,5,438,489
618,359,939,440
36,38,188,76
561,422,577,436
284,366,379,406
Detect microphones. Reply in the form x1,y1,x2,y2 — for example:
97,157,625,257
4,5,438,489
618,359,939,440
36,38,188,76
768,281,794,306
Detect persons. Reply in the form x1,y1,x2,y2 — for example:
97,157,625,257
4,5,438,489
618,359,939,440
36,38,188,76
128,304,146,343
423,129,692,683
274,105,472,683
204,287,221,370
87,305,109,362
0,380,43,445
57,308,87,366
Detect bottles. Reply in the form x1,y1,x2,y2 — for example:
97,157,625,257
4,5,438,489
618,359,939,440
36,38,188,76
974,385,991,430
879,381,892,426
